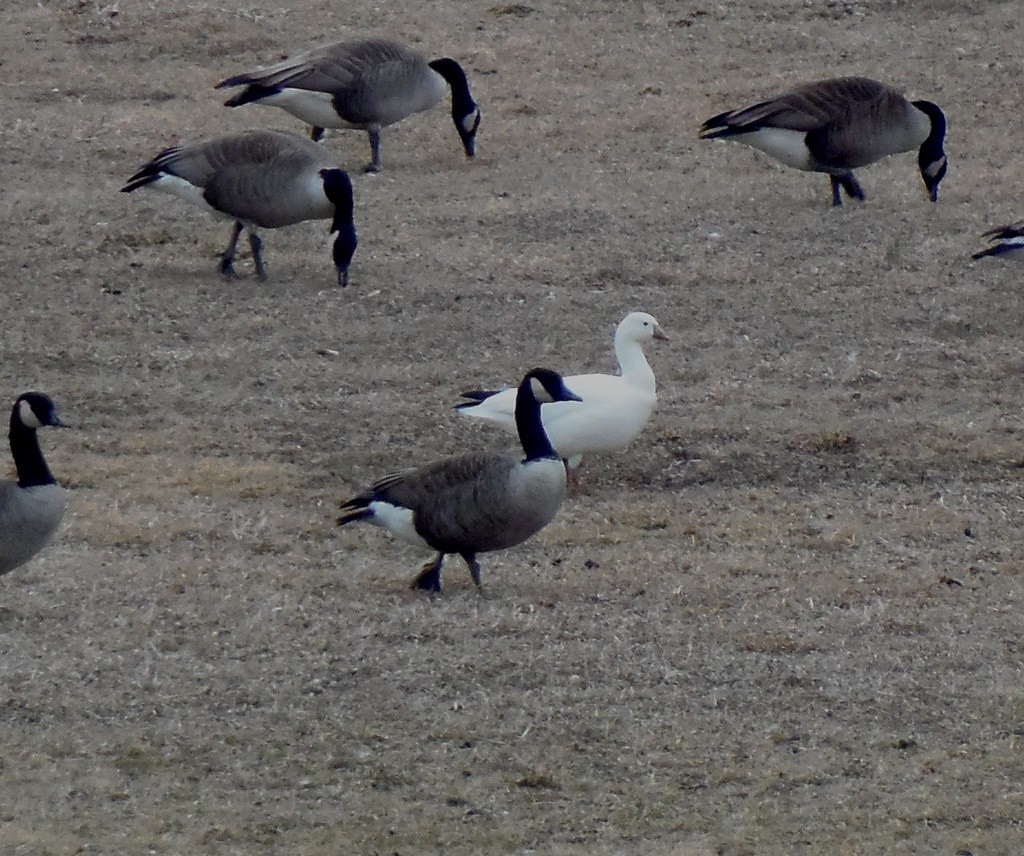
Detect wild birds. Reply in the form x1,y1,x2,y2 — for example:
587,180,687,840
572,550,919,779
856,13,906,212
697,74,947,208
213,36,480,175
337,366,583,599
0,392,72,583
450,312,668,494
120,128,356,287
972,220,1024,264
